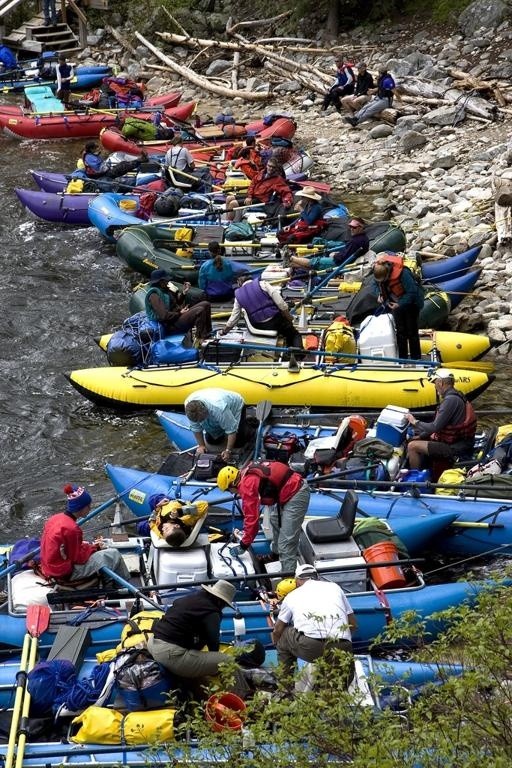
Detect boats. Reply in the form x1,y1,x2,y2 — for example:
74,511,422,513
0,512,512,661
0,52,487,335
106,409,512,558
61,322,499,415
0,649,494,768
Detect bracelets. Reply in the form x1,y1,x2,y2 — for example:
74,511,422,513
225,449,234,455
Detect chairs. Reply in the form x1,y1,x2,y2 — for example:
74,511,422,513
236,302,279,339
451,425,500,471
304,486,359,544
167,167,200,192
288,415,355,475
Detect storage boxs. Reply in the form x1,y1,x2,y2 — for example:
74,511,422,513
206,340,241,363
137,168,163,180
354,310,397,366
224,236,255,258
148,539,209,600
375,404,415,447
191,223,224,247
261,236,280,252
207,536,254,600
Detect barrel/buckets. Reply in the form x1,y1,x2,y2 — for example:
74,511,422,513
348,414,367,441
67,179,84,193
364,541,406,590
206,693,248,733
174,228,191,241
120,199,137,216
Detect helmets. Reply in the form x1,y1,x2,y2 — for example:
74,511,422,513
277,578,297,599
217,465,239,492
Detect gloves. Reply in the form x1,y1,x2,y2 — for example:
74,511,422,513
230,545,245,557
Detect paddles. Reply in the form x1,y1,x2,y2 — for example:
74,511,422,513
254,399,271,461
105,201,277,236
0,475,152,580
205,507,504,530
0,80,57,93
153,239,325,250
218,342,495,374
15,605,51,768
26,105,165,113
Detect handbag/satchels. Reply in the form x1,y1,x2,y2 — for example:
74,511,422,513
122,116,157,140
193,452,223,480
156,186,182,216
263,430,299,462
326,320,357,363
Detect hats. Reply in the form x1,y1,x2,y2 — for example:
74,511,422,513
295,564,318,579
150,268,173,285
64,484,92,511
294,185,322,202
434,368,454,379
202,579,238,611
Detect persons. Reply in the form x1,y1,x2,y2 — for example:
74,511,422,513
271,564,357,712
56,53,75,102
316,53,355,113
341,62,373,118
185,388,250,463
43,0,57,27
37,111,427,367
137,493,209,547
345,65,405,127
1,44,17,79
491,175,512,249
404,368,477,473
40,485,131,590
148,580,251,698
216,458,311,580
